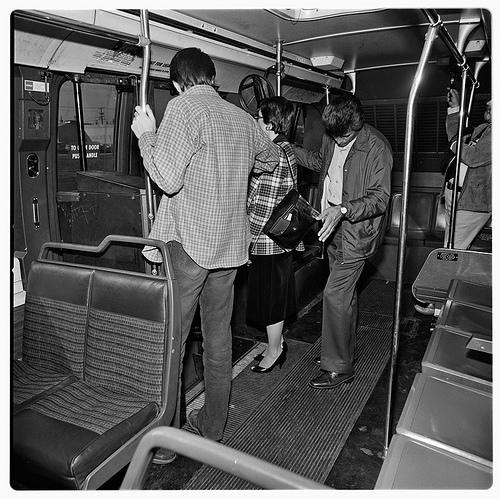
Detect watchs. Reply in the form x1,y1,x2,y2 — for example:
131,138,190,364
337,203,348,221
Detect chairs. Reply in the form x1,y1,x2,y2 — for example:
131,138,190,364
421,326,492,387
395,373,493,467
437,300,492,340
10,235,181,489
384,192,448,247
448,278,491,307
413,247,494,317
374,434,492,489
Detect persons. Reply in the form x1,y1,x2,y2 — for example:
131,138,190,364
129,47,281,465
245,94,308,375
413,86,492,316
287,93,395,390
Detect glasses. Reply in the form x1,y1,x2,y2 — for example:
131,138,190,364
253,116,263,120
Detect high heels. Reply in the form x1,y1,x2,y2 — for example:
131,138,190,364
250,340,288,373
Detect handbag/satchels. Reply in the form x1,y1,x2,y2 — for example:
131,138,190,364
262,188,322,250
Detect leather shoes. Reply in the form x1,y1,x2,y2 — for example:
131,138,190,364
187,409,206,437
308,352,355,389
153,450,176,464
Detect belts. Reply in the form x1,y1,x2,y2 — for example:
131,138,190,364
446,182,462,193
327,200,338,209
